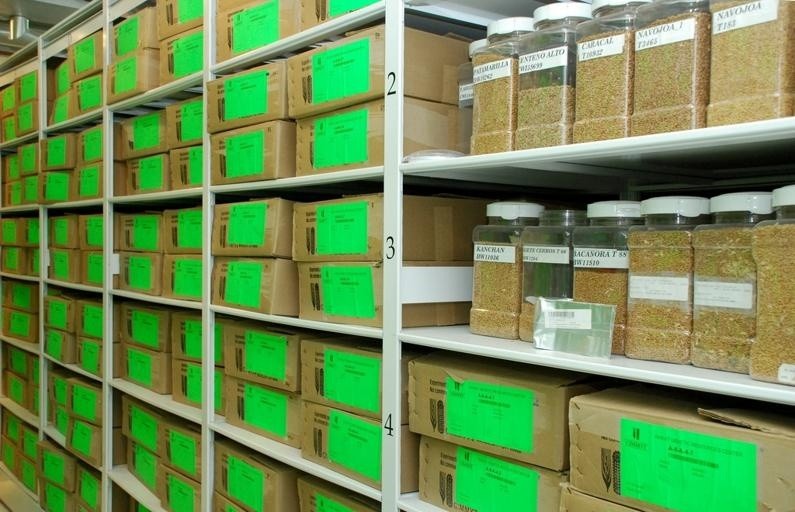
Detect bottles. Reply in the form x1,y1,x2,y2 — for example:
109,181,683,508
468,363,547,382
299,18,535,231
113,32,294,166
454,0,794,156
466,186,795,389
